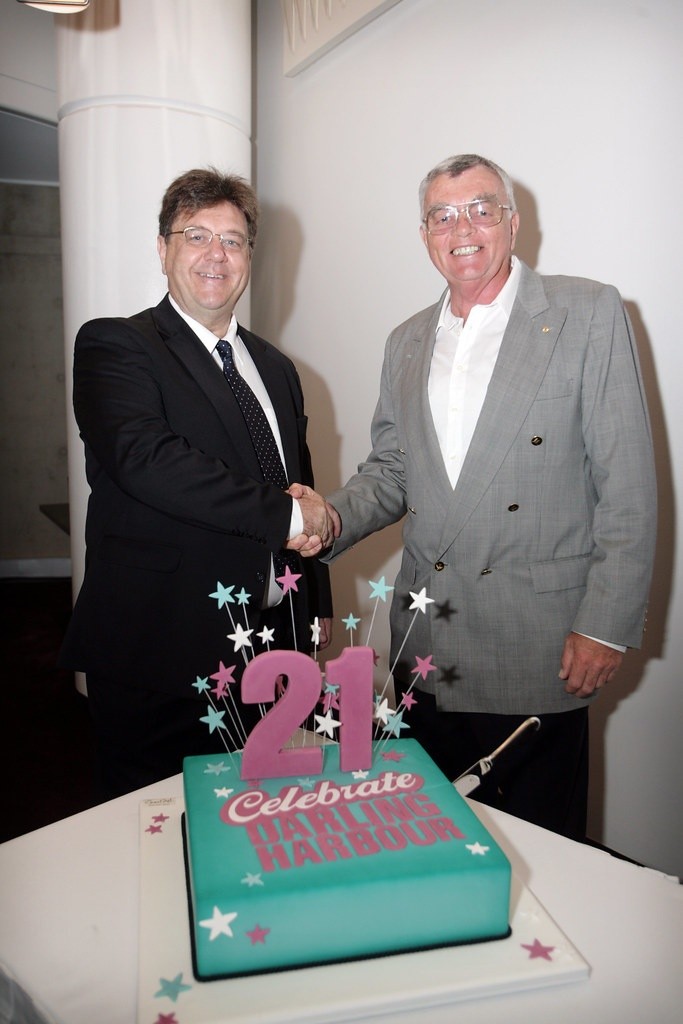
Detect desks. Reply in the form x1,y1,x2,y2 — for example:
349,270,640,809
0,721,683,1024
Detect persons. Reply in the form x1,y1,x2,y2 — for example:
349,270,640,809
54,167,333,813
285,154,659,847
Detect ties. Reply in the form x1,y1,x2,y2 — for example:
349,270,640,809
216,338,299,597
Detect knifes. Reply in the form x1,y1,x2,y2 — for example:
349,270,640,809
452,717,540,799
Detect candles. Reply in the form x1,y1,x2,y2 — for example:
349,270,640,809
241,649,324,780
326,647,374,771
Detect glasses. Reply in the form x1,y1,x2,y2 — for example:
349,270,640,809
422,200,512,235
164,226,254,254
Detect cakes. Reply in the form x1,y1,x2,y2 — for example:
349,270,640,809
183,738,510,978
